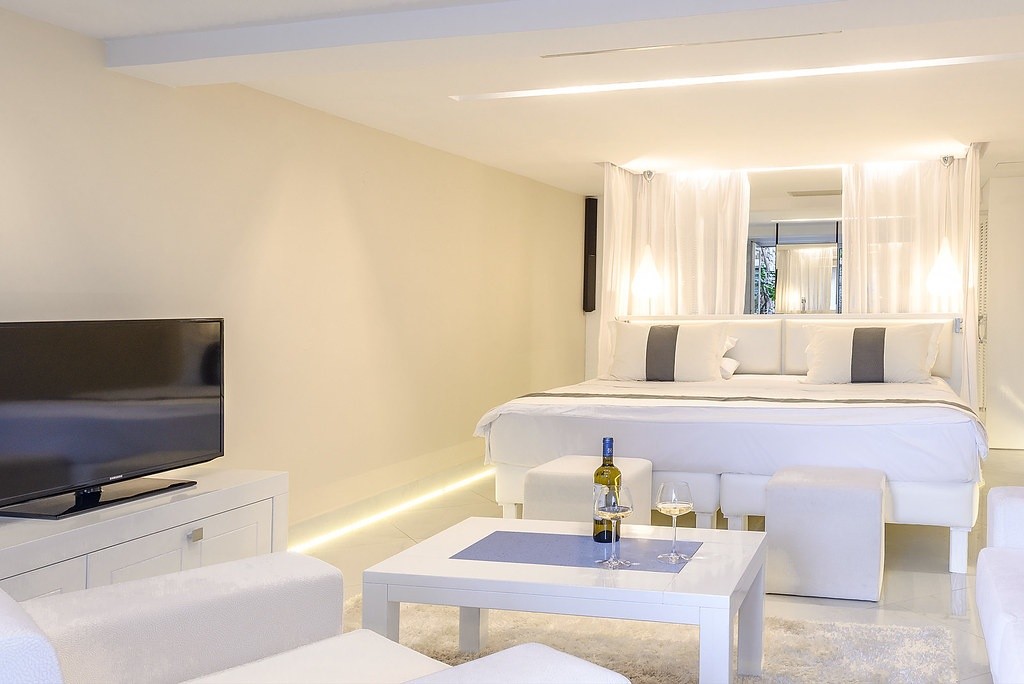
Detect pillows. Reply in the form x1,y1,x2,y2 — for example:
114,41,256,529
798,324,944,384
607,321,728,380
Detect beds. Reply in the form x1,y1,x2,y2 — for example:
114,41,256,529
473,314,989,574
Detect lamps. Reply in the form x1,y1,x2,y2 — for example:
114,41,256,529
920,157,967,290
620,170,673,296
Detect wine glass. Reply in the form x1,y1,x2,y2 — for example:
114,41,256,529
593,485,633,570
656,480,695,564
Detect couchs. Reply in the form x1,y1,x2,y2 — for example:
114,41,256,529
976,486,1024,684
0,547,632,684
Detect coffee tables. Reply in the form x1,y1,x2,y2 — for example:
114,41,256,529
362,517,768,684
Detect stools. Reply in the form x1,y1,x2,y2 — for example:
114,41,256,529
523,455,652,525
764,467,886,602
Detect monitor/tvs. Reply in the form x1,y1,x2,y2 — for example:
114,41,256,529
0,317,224,519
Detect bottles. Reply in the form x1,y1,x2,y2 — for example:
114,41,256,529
593,437,622,543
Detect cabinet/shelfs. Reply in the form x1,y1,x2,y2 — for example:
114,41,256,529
977,177,1024,449
0,467,287,602
